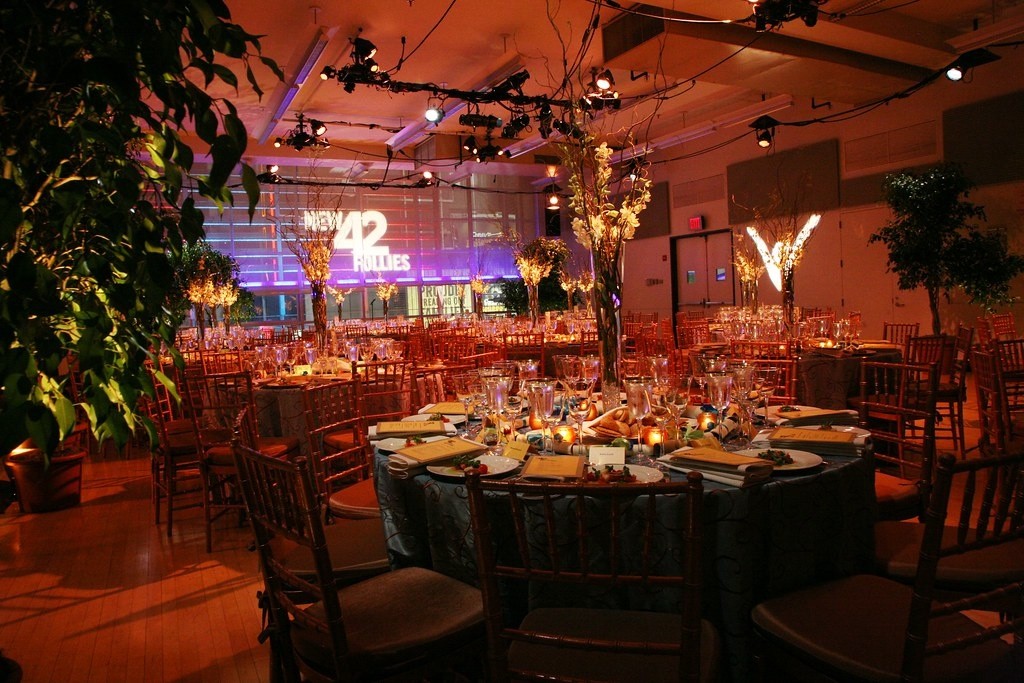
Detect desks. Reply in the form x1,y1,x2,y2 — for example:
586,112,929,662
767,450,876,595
687,337,901,411
373,394,880,682
217,370,381,475
474,334,633,375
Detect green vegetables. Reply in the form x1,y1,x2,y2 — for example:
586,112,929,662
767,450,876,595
592,464,631,483
821,418,833,430
427,411,444,422
406,435,423,444
452,454,481,468
758,449,794,465
781,404,799,412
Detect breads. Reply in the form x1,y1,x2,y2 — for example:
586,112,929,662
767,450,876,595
589,407,678,438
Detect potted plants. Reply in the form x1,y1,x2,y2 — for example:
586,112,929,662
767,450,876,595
866,160,1024,376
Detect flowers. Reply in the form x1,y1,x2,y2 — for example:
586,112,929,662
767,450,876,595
513,0,677,384
178,147,594,343
728,157,825,324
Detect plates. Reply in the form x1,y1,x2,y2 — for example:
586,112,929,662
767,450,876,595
375,436,451,452
591,392,627,401
401,414,466,425
582,464,664,483
754,405,822,417
734,449,823,470
795,425,871,438
508,395,521,403
426,454,519,478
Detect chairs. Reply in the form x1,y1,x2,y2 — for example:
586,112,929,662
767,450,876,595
68,307,1024,683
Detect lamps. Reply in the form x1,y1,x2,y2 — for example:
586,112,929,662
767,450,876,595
948,54,975,81
255,0,819,206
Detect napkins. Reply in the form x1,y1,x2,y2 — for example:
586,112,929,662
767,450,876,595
387,454,440,479
657,445,772,488
769,435,866,456
367,425,422,440
766,409,858,426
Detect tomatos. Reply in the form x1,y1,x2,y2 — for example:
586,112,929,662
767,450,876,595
478,464,488,474
587,471,595,481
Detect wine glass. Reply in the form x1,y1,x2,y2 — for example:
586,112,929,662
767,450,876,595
366,318,410,335
478,317,533,344
452,355,540,456
245,341,338,378
623,355,693,472
326,320,366,335
203,323,263,354
689,355,780,454
714,305,784,344
786,320,850,350
436,313,478,335
524,355,600,464
343,338,405,375
160,328,199,360
537,311,598,343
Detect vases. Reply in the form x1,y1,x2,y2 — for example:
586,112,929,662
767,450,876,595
382,299,388,322
475,291,484,321
743,280,758,316
5,445,87,514
527,284,538,330
567,289,572,313
337,303,342,323
588,241,625,414
209,305,219,328
310,280,327,352
780,270,795,323
224,305,230,335
193,303,204,341
